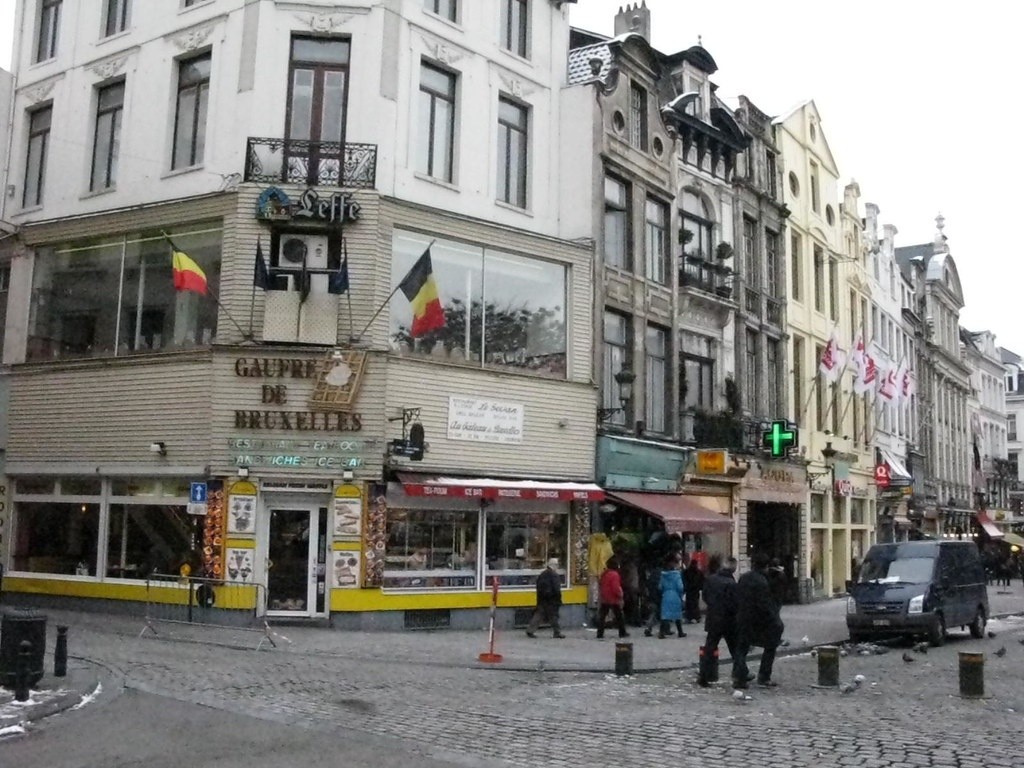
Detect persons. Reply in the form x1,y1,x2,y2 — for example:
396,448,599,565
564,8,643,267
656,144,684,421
526,533,720,639
464,542,477,569
696,557,785,688
956,523,964,540
973,524,985,554
133,544,204,581
408,547,430,570
984,548,1024,586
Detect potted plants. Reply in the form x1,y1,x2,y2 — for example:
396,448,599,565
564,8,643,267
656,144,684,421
680,230,733,297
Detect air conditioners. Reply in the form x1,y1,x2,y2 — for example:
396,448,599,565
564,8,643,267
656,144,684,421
280,234,328,268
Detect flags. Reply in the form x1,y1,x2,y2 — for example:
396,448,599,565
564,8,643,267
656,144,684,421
172,249,208,297
400,249,446,337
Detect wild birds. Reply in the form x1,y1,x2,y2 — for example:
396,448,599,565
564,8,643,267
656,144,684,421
732,631,1024,706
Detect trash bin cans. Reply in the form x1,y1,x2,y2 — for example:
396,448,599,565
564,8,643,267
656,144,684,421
0,607,48,687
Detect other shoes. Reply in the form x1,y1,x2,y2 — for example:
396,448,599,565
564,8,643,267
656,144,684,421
553,634,565,638
664,631,674,635
645,631,652,637
596,635,605,638
747,673,756,680
678,632,686,637
619,633,629,638
697,678,710,688
757,679,777,686
732,680,749,689
526,632,536,638
658,634,666,639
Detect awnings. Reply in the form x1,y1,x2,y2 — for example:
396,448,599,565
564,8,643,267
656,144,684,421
605,490,735,535
396,471,605,502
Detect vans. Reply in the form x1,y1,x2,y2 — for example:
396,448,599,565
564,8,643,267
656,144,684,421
844,539,992,648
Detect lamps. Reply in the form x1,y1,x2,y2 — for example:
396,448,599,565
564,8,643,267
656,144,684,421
588,58,602,75
343,468,354,483
151,441,166,455
600,362,634,418
807,442,837,479
237,465,251,478
